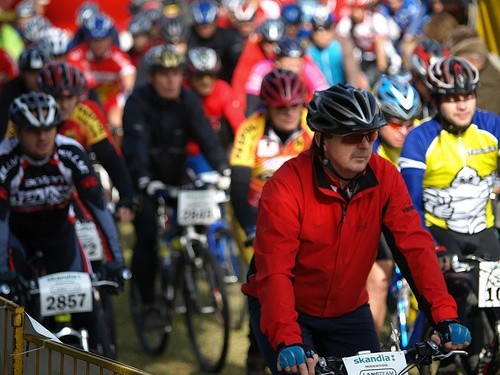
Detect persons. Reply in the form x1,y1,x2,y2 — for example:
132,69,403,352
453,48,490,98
0,0,500,375
241,81,473,374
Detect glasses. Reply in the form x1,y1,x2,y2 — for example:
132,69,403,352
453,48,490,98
386,119,414,132
339,131,378,144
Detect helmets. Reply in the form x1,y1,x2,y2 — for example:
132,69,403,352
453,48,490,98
424,56,479,95
411,39,450,75
307,82,389,136
9,92,61,132
15,0,335,97
259,68,305,106
374,74,422,120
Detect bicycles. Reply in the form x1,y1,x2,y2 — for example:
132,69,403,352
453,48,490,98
0,187,130,360
284,338,469,374
434,253,500,375
135,184,230,375
